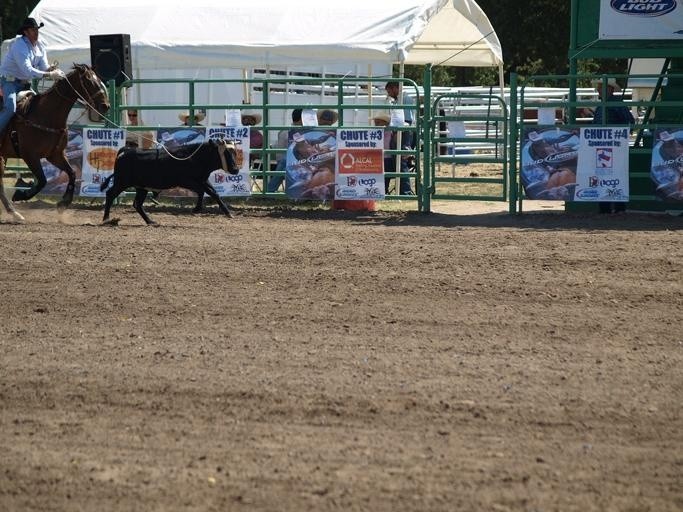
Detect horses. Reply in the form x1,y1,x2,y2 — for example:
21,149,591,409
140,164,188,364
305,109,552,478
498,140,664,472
0,61,112,222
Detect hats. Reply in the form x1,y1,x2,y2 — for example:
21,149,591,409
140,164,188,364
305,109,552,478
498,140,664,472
179,108,205,122
367,111,391,124
17,18,45,35
590,77,622,92
316,109,339,124
239,108,262,124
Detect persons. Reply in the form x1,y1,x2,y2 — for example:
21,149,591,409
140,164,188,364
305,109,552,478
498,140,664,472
590,75,634,213
178,109,205,126
267,107,302,192
369,110,393,195
0,16,65,138
385,81,416,195
126,109,153,151
402,91,414,125
240,108,263,163
315,109,338,126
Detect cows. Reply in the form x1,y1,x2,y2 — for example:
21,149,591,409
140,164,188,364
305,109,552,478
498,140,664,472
301,166,339,200
99,139,239,225
535,168,580,200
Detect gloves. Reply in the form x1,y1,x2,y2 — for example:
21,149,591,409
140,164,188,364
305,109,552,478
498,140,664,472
50,69,65,81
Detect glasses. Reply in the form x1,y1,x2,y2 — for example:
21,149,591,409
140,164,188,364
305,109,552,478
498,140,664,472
128,114,138,118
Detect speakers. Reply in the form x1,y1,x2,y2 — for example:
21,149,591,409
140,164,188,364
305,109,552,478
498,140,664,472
89,34,133,88
